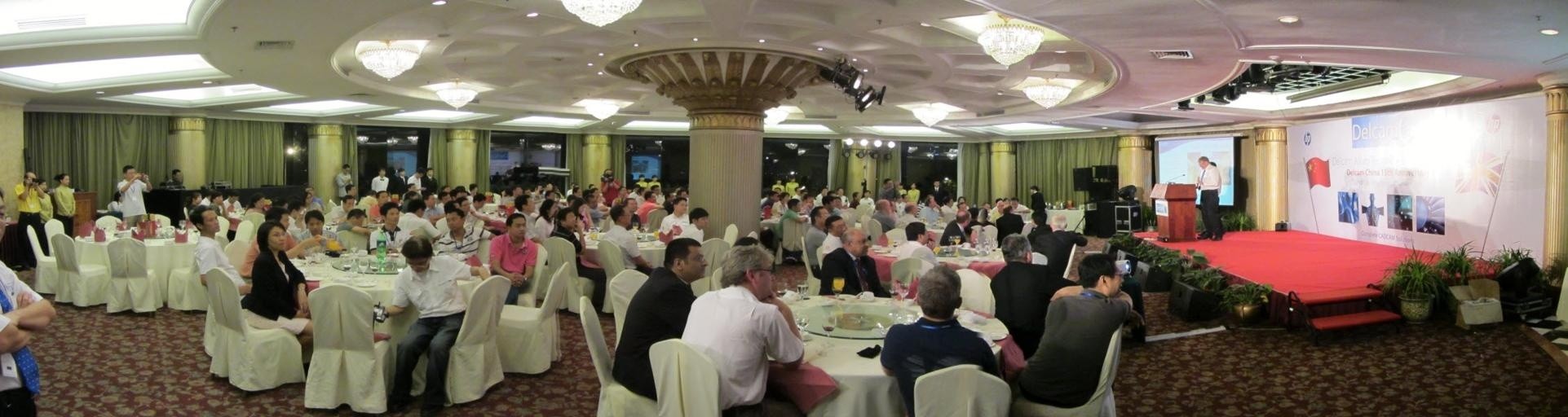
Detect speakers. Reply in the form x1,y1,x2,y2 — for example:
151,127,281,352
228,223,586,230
1167,279,1225,322
1116,249,1140,276
1133,259,1173,292
1073,167,1093,191
1102,241,1128,255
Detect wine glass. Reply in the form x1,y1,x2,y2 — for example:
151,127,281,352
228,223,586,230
293,248,378,284
98,217,188,242
586,221,649,243
932,232,999,260
773,276,909,339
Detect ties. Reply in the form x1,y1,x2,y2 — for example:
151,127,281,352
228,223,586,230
0,289,40,395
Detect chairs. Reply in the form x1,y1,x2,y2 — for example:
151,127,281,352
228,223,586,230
1062,244,1076,280
578,294,655,415
205,268,304,389
691,276,717,298
304,284,393,415
50,232,105,308
609,269,649,349
756,190,1085,241
724,224,739,246
234,220,254,243
597,239,626,314
544,237,593,314
891,258,934,298
183,206,189,223
747,231,757,241
150,213,171,228
95,215,122,233
799,236,821,295
956,268,996,319
104,237,163,313
410,274,512,405
911,365,1012,417
500,261,569,375
169,239,246,313
517,243,548,306
26,224,58,295
648,339,722,415
44,219,65,255
215,215,230,236
1016,326,1124,417
336,230,367,252
699,237,731,277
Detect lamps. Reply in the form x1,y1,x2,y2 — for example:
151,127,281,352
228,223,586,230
840,138,895,160
1172,63,1329,111
353,0,1083,129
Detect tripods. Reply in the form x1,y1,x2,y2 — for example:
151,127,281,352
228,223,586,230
1073,191,1087,236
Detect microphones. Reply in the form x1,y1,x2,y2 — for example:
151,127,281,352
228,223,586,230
1166,174,1185,183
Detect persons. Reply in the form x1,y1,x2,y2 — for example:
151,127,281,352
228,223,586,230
1197,156,1224,241
1,164,1146,417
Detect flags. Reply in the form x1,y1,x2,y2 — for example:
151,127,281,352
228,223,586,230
1455,151,1506,198
1305,157,1332,189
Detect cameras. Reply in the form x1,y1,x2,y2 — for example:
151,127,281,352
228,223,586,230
373,305,389,323
32,178,45,185
139,174,144,178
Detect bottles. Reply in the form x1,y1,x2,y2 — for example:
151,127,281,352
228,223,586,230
376,230,386,260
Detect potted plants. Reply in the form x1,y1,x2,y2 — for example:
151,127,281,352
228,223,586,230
1378,239,1438,324
1434,240,1482,313
1214,283,1273,325
1142,201,1157,232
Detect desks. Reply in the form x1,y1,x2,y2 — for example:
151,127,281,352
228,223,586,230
190,188,684,227
577,231,689,267
778,293,1011,417
74,230,211,289
287,252,408,297
863,241,1045,273
